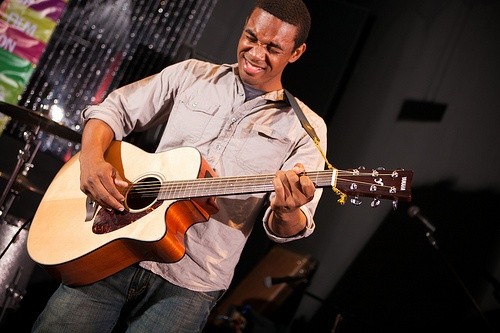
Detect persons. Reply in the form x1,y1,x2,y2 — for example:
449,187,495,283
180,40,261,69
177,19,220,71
29,0,327,333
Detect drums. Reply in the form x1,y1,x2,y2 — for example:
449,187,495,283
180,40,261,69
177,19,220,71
0,210,38,324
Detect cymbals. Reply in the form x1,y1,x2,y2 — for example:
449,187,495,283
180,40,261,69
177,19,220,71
0,173,44,197
0,101,82,145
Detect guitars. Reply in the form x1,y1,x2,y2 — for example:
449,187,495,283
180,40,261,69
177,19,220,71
27,139,414,289
